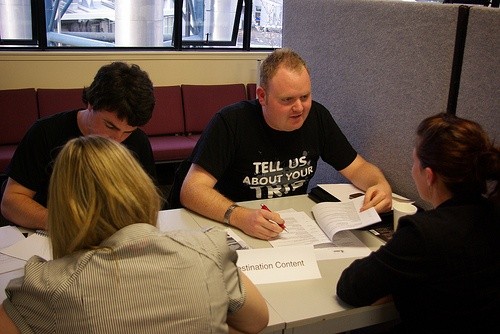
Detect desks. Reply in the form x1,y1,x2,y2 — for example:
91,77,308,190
0,194,402,334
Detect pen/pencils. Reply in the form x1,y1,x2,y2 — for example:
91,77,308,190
261,204,289,233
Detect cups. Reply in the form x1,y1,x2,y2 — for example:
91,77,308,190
394,202,418,232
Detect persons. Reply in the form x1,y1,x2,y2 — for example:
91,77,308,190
180,47,392,240
0,135,270,334
336,112,500,334
1,61,156,233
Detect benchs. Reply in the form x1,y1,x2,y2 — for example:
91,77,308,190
0,83,256,209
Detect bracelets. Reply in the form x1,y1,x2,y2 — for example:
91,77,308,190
223,204,238,225
